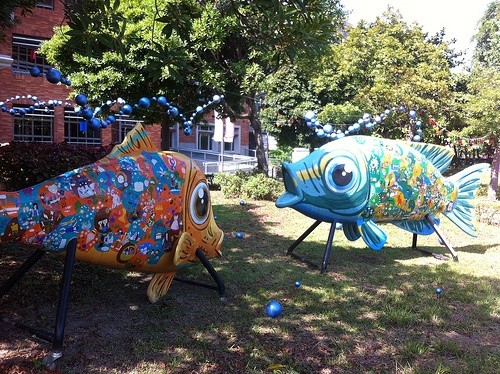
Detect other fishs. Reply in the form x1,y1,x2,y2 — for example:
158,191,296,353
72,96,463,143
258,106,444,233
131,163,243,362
274,135,490,251
0,122,224,304
